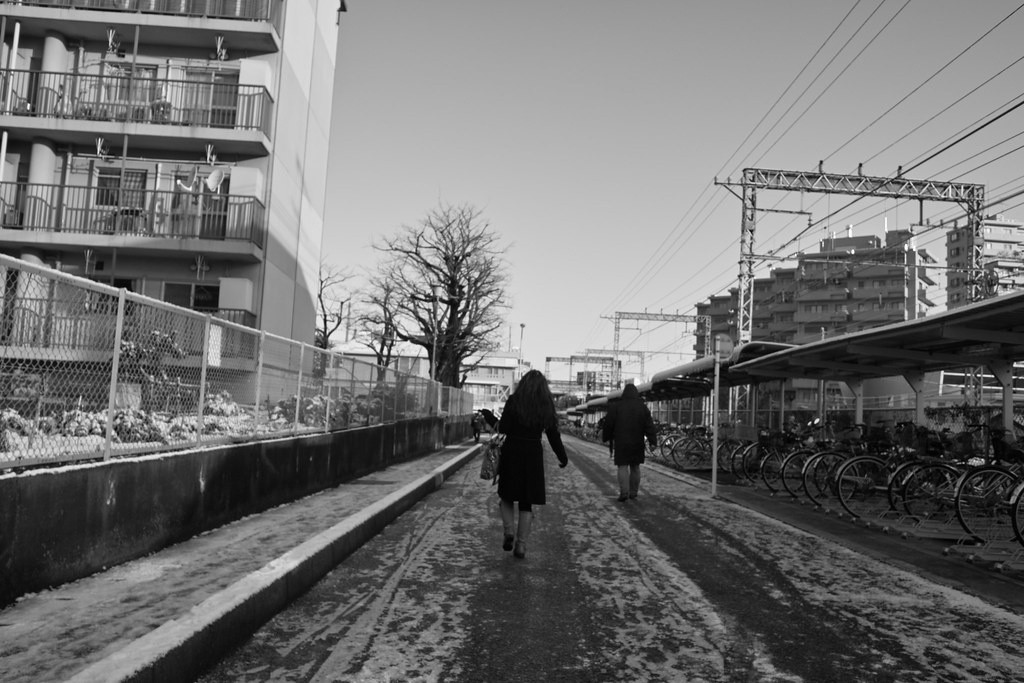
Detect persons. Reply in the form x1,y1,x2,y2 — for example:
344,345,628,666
602,383,657,502
471,413,484,443
481,370,568,558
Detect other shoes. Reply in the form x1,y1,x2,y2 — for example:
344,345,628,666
629,494,637,498
617,493,629,501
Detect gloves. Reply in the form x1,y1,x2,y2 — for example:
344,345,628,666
558,458,568,468
478,409,491,415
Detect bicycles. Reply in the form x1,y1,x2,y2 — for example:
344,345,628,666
644,416,1024,548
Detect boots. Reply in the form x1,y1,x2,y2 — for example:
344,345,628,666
513,511,533,559
499,499,514,551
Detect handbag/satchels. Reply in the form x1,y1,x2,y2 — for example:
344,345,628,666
480,422,502,479
